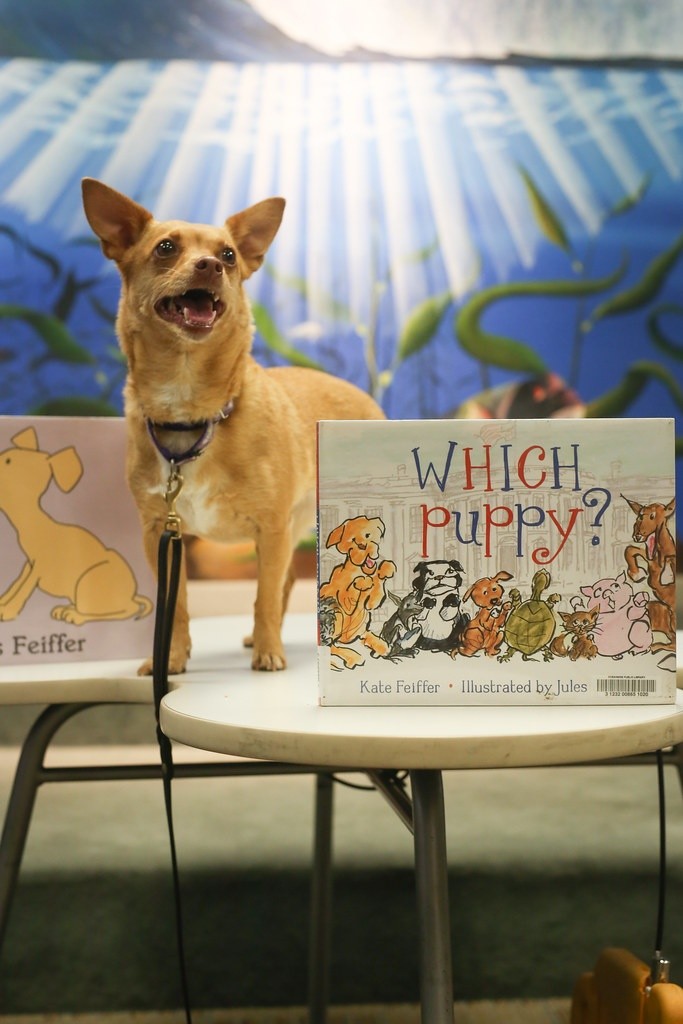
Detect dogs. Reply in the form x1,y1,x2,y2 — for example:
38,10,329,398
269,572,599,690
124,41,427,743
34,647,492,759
81,177,390,675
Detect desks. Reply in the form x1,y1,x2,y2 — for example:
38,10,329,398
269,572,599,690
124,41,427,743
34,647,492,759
0,611,683,1024
